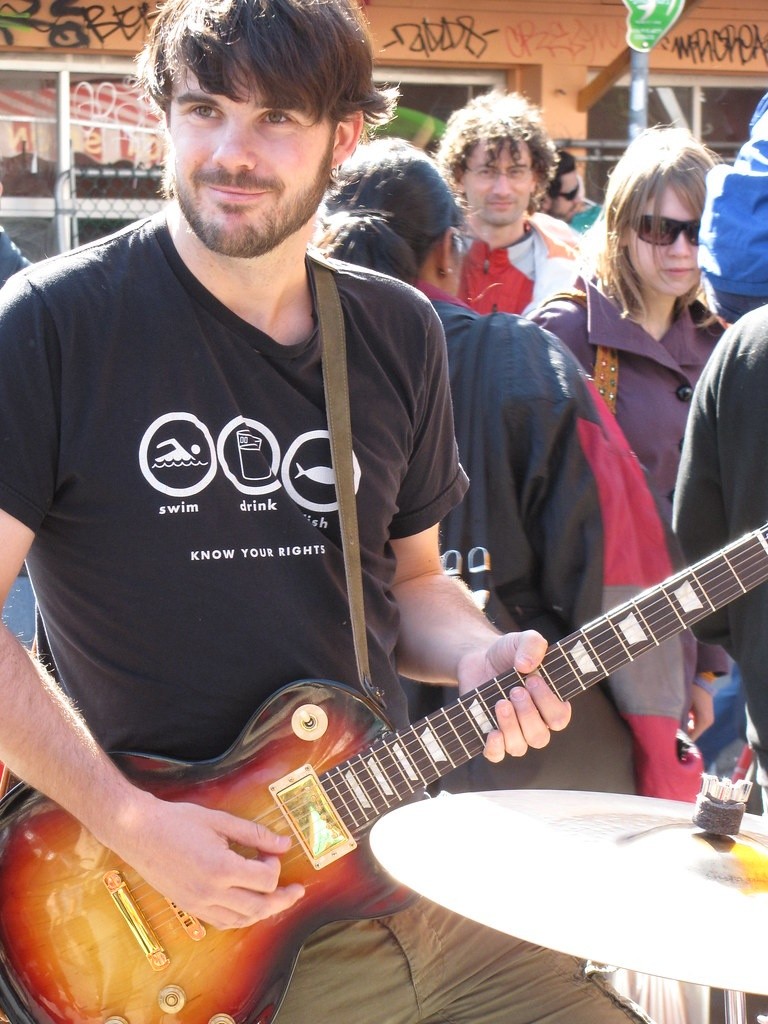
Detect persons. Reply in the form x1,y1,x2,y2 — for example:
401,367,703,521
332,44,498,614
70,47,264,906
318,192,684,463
0,1,649,1024
308,81,767,804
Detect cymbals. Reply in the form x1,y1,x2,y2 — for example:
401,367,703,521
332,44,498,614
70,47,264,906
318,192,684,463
367,787,768,996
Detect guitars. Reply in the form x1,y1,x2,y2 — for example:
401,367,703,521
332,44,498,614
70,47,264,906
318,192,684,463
2,522,768,1024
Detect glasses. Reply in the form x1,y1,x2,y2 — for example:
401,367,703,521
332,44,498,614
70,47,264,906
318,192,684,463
629,215,700,246
